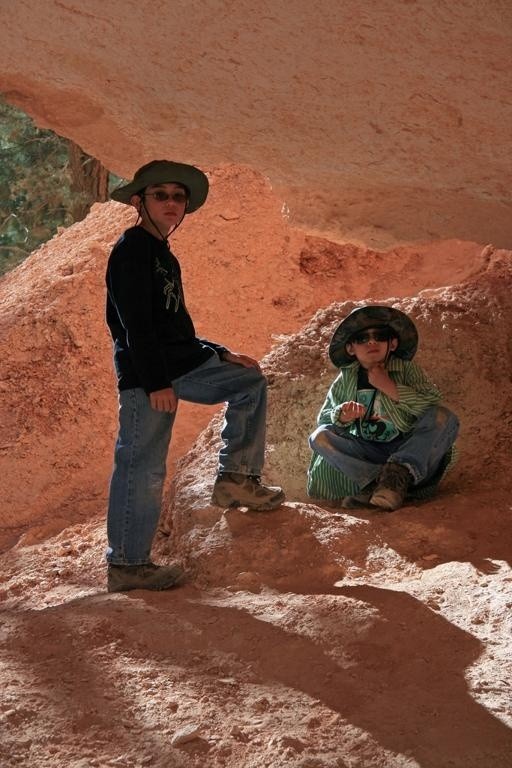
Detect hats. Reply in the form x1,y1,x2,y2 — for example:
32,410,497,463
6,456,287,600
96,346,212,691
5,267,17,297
329,305,418,369
110,159,209,214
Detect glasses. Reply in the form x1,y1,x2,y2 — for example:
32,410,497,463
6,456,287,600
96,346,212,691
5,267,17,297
352,330,391,345
145,191,188,203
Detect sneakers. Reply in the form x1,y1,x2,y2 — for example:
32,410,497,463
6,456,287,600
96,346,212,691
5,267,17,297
210,472,285,512
341,494,368,510
107,562,184,594
369,461,412,510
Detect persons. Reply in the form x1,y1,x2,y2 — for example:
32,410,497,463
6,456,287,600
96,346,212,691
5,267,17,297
105,159,287,594
306,301,461,511
